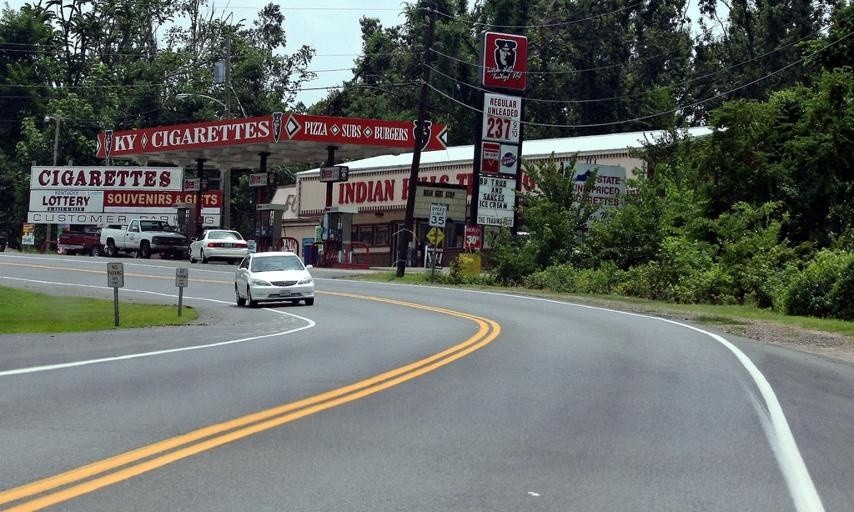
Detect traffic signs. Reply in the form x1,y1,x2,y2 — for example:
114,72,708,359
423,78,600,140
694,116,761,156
428,203,447,229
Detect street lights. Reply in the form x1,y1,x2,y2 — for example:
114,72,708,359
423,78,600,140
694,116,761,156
42,112,62,250
174,91,235,228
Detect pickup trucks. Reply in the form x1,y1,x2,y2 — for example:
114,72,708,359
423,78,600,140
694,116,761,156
98,216,188,260
56,227,107,257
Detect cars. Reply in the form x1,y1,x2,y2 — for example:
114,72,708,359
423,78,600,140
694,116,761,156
229,251,316,310
187,228,249,264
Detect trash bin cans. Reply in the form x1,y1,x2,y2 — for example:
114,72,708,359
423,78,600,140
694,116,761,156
303,244,318,268
